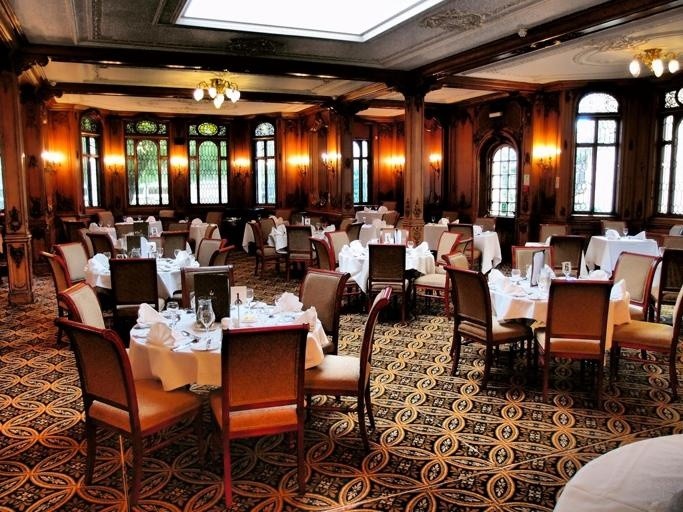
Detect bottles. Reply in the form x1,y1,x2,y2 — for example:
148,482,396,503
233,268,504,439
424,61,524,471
195,297,215,329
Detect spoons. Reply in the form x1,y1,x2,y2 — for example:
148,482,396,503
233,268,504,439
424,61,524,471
169,330,200,352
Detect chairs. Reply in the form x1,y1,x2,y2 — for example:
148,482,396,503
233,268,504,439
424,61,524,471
36,206,394,505
242,205,682,399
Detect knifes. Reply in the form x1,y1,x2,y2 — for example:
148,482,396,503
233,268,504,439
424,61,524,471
205,338,211,349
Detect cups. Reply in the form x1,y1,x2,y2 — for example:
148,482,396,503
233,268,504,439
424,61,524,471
511,269,520,279
537,272,549,290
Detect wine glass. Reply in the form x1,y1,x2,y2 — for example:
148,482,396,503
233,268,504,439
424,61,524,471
98,221,111,230
246,289,254,306
561,262,572,279
622,227,628,238
369,205,378,211
198,310,213,335
102,247,180,262
315,222,320,233
432,217,435,224
407,240,413,249
362,216,367,224
122,215,141,223
166,302,179,323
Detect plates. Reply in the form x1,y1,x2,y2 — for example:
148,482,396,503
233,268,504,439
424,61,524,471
192,342,219,351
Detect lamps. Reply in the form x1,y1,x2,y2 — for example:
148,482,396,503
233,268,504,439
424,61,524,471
627,48,679,79
191,78,241,108
